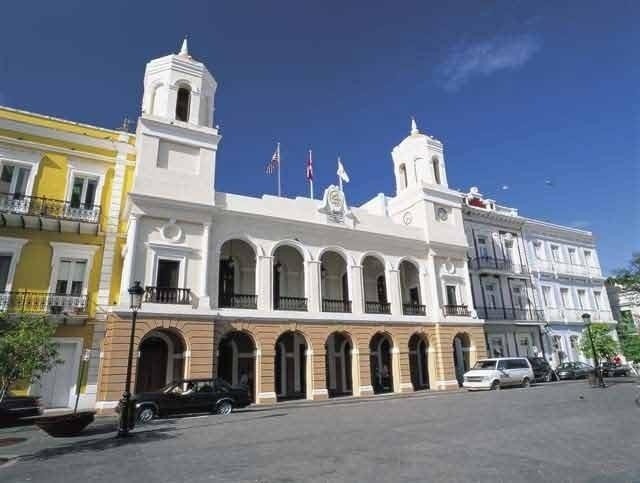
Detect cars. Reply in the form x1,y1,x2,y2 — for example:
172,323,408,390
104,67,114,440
602,363,633,377
554,361,595,379
117,377,251,424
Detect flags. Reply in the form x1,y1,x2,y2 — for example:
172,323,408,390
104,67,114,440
265,150,278,176
336,157,349,183
306,152,313,181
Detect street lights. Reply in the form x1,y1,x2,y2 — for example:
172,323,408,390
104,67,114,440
582,313,608,387
117,280,145,435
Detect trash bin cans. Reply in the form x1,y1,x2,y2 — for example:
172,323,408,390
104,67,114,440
587,369,601,388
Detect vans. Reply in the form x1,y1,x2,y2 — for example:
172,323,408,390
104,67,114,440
462,357,535,390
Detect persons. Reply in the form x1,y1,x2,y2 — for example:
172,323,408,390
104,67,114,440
178,384,192,396
612,355,620,364
